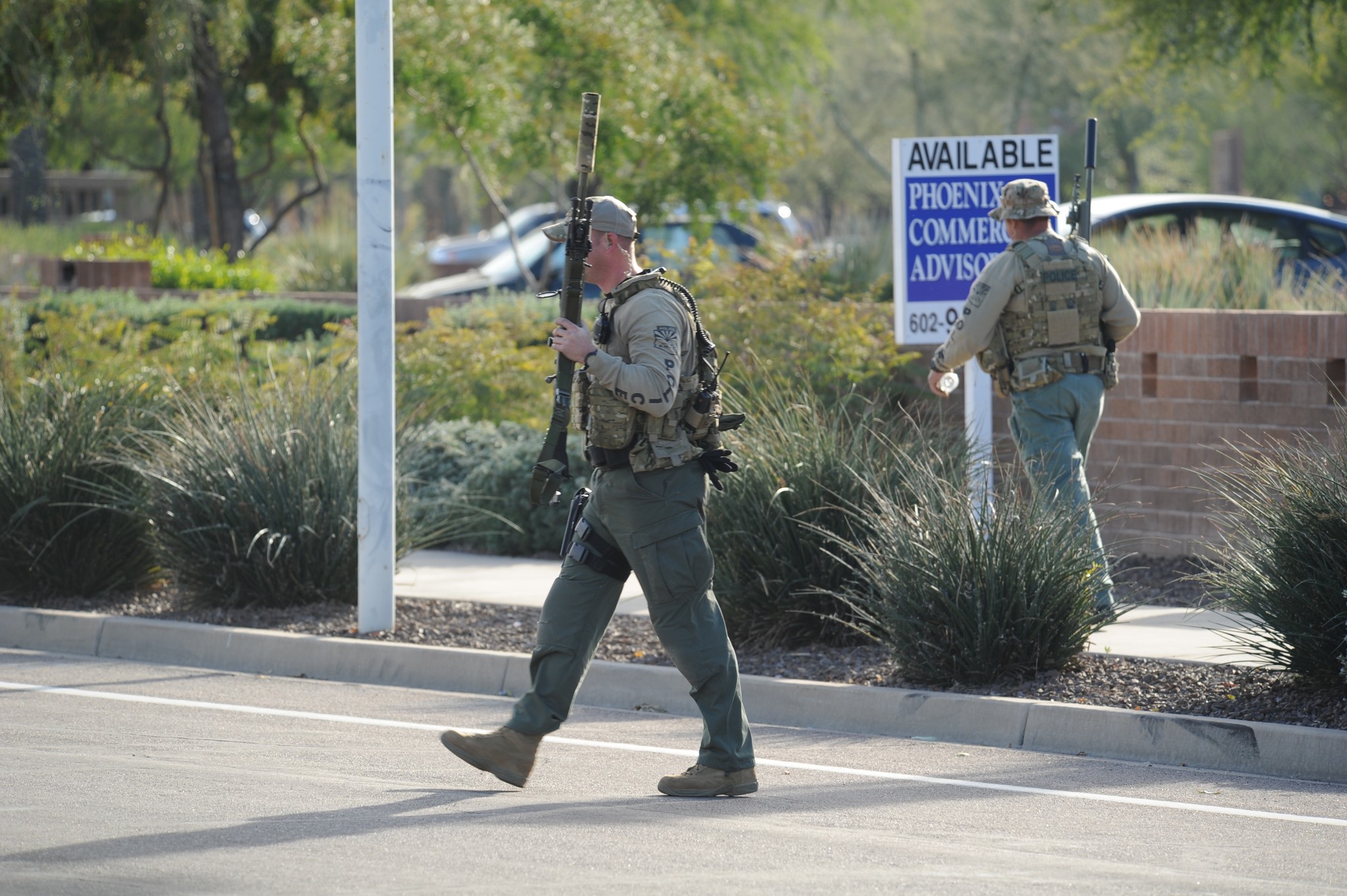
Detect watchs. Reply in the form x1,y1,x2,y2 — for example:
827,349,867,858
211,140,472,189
583,348,598,370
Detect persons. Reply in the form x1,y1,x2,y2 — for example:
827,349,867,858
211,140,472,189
926,176,1142,624
439,193,762,801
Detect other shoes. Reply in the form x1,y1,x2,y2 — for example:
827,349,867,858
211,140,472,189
1090,605,1116,623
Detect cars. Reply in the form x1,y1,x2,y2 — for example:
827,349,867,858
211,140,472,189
401,197,805,324
1049,192,1347,290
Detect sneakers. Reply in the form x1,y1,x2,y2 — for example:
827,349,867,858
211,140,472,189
442,729,544,787
658,764,759,796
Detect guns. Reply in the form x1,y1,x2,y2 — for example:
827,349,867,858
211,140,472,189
1068,117,1119,388
528,91,600,509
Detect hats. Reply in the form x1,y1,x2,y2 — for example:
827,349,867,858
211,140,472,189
989,179,1059,219
542,195,640,242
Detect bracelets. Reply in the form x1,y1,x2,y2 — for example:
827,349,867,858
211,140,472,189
929,359,944,374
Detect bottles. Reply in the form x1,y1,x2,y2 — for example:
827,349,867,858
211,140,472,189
935,372,960,393
